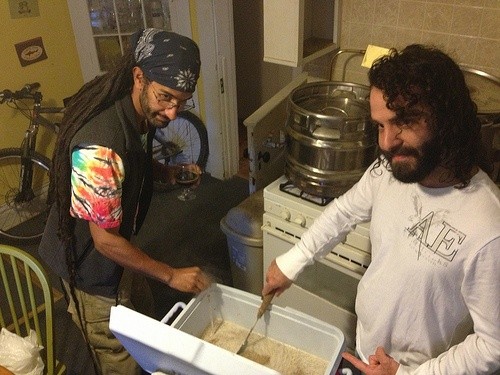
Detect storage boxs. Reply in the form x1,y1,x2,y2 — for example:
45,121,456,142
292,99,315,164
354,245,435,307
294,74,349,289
109,283,345,375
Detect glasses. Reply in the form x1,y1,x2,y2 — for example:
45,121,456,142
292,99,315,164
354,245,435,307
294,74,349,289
149,84,196,114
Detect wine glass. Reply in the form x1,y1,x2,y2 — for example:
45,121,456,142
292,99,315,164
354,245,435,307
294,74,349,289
176,160,198,201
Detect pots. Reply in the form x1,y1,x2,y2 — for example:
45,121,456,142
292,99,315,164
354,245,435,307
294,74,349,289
459,65,500,186
280,81,380,197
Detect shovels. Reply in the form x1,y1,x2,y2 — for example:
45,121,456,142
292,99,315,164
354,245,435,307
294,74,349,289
235,287,277,355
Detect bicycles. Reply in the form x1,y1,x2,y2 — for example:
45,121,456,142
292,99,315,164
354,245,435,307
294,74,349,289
0,80,209,248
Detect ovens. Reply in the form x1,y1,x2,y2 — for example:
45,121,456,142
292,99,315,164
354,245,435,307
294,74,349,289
261,211,372,358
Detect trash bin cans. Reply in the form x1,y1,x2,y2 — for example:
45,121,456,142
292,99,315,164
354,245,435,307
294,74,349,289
219,187,263,298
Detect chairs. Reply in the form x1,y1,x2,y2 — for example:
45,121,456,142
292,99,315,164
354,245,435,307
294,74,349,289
0,243,55,375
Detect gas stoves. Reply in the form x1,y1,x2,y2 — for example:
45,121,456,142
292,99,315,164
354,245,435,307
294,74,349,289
263,175,371,253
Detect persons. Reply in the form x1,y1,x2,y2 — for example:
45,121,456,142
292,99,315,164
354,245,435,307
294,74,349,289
39,25,213,375
261,43,500,375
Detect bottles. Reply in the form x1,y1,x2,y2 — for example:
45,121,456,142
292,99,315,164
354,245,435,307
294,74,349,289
149,0,165,30
